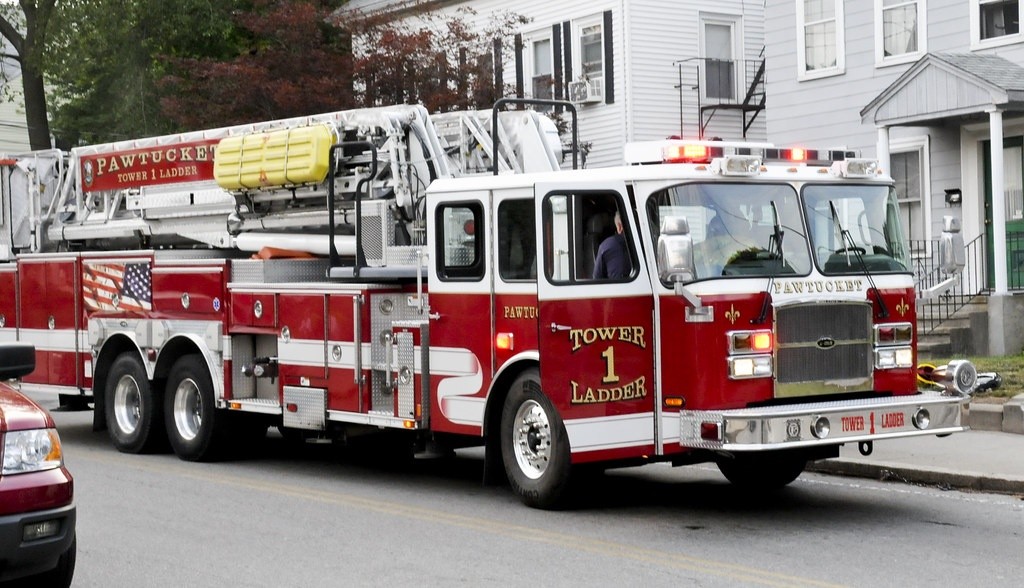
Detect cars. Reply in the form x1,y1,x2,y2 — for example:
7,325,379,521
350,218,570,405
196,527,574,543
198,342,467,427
0,345,78,588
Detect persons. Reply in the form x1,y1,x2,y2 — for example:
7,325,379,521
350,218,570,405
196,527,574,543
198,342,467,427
593,211,631,279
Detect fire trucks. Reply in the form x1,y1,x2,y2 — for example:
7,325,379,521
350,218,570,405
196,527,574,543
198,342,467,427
0,98,976,510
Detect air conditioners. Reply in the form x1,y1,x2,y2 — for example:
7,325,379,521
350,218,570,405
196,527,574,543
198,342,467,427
568,79,602,104
